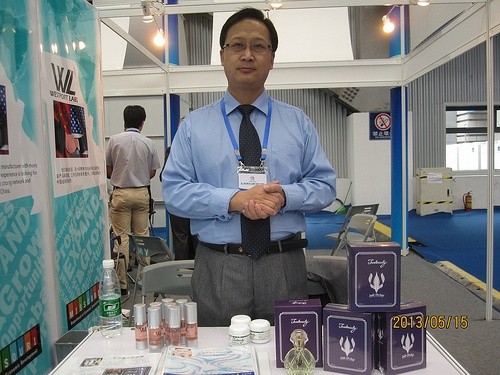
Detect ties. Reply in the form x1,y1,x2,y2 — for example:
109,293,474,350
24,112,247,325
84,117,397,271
238,104,272,261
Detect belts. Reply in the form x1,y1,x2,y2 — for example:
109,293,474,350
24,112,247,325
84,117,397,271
199,234,308,254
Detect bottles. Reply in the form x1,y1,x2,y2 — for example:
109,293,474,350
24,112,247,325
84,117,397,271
229,323,250,345
251,318,271,343
283,328,315,375
98,258,123,337
230,314,251,326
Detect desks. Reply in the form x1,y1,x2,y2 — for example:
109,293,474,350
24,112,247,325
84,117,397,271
48,324,468,375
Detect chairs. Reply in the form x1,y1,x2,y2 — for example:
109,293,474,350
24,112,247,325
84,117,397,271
110,232,195,326
325,204,380,257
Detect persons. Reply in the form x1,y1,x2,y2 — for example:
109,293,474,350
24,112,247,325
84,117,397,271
159,116,198,261
53,100,87,158
162,8,336,327
106,105,161,295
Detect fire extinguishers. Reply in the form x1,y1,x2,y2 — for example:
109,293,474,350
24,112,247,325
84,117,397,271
463,190,473,212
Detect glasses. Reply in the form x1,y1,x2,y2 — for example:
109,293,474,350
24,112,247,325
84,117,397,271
223,39,274,53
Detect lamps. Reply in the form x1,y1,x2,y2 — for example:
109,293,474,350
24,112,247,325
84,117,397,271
382,6,396,32
141,0,154,23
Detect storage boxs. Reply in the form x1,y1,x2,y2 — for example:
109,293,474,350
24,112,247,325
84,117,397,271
274,242,426,375
